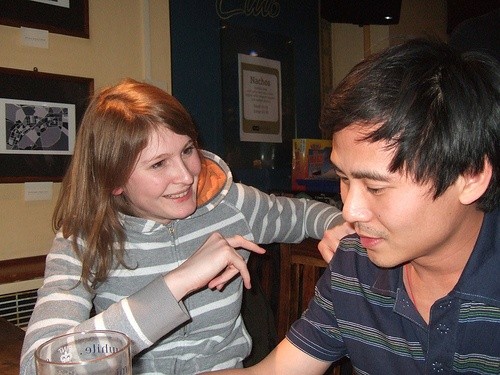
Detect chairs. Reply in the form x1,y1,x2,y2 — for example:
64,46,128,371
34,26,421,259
275,239,358,375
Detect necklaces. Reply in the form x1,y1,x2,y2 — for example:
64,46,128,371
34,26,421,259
404,263,418,311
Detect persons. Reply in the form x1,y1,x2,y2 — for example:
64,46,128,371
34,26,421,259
187,39,498,375
20,79,356,375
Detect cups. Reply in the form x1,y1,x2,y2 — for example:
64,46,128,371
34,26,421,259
34,330,132,375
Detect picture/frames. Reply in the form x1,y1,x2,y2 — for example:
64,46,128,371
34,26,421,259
1,67,95,183
1,0,91,39
